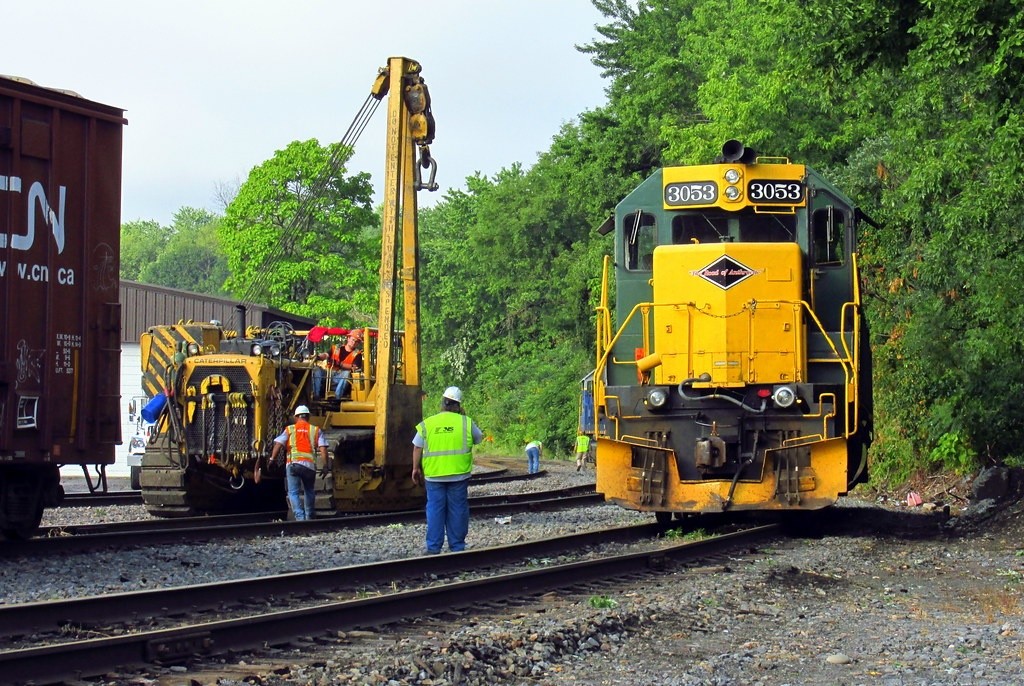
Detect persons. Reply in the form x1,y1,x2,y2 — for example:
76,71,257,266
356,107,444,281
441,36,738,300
267,405,329,525
574,433,591,471
411,385,487,555
319,329,365,403
524,438,543,477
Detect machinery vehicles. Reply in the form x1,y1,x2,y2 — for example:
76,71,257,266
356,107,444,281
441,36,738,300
137,56,438,534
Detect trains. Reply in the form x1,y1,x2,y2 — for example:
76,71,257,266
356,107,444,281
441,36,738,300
576,138,884,527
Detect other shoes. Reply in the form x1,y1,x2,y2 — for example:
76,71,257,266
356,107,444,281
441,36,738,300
576,465,581,471
331,398,341,405
582,466,587,471
313,395,324,401
424,551,439,556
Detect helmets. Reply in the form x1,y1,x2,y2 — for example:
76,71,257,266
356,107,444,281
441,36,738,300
580,431,585,435
347,329,364,341
294,404,310,417
443,386,462,403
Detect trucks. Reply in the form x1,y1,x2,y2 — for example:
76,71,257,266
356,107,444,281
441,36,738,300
0,80,130,566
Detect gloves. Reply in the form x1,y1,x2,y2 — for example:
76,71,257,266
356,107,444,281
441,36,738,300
266,457,278,473
319,465,329,480
